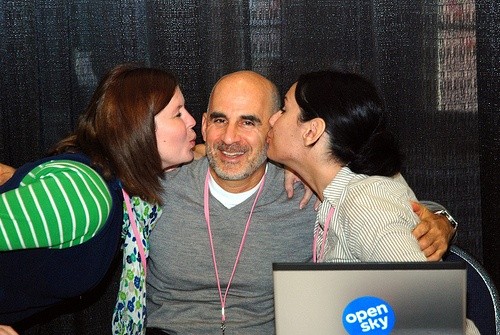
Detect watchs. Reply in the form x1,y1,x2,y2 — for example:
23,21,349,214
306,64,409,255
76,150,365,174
435,209,459,243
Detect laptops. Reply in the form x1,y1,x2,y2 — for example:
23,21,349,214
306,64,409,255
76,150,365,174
272,261,467,335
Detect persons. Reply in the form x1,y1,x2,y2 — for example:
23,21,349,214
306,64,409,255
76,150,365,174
1,64,319,335
191,69,483,334
0,71,482,335
